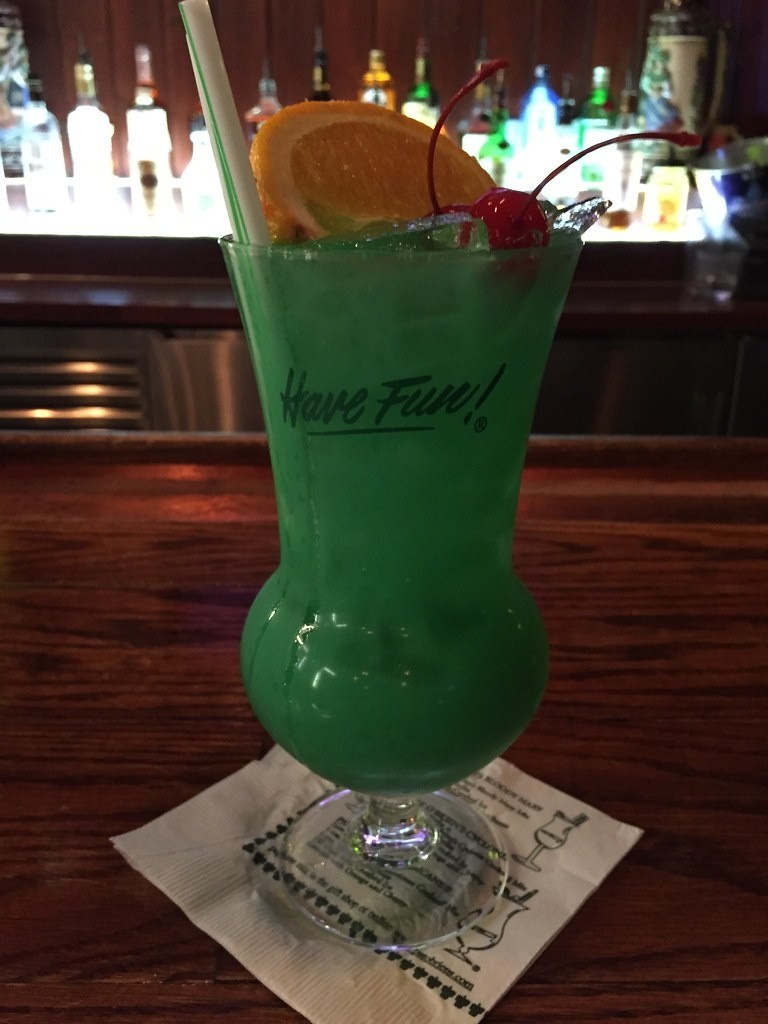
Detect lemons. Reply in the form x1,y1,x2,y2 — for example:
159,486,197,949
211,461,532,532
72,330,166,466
247,101,501,246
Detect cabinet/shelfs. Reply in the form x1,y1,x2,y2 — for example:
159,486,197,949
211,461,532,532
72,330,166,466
0,178,767,436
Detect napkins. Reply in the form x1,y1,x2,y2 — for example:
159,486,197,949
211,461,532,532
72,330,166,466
109,742,646,1024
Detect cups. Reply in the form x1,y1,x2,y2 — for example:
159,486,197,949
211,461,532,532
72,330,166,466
643,167,690,231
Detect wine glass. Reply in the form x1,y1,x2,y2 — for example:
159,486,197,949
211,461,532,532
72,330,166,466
221,232,582,950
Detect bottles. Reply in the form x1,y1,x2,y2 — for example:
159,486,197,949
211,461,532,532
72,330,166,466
401,39,444,135
22,77,69,212
519,64,559,149
180,118,229,223
67,63,116,209
306,50,335,102
127,45,177,220
571,66,620,184
596,90,643,231
457,59,501,184
244,77,284,154
359,49,396,112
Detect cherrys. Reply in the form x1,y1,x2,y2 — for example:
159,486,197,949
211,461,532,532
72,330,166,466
419,58,703,271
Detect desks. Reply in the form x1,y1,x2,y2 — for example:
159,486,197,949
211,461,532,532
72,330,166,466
0,429,768,1024
